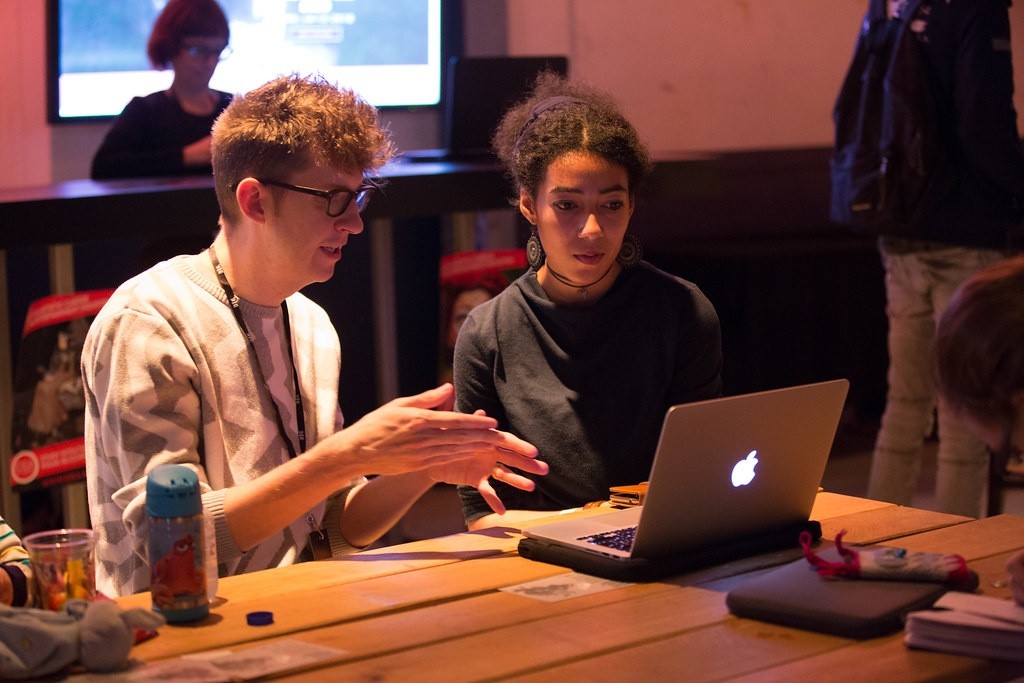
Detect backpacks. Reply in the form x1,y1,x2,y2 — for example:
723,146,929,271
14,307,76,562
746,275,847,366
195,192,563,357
829,0,941,244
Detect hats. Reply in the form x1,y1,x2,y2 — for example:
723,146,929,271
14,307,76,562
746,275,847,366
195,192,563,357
436,248,529,298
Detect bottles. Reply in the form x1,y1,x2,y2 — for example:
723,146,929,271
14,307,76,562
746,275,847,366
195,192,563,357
175,462,219,604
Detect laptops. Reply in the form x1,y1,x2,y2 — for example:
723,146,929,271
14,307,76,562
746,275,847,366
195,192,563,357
521,379,850,560
404,54,570,162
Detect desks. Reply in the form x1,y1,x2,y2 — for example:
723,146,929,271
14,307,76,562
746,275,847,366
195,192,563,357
1,148,520,403
0,485,1024,683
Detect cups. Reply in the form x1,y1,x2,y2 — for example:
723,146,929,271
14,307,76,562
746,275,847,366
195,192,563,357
144,463,209,625
21,528,97,613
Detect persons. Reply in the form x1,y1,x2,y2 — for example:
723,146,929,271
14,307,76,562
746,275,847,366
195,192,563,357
867,0,1024,520
91,0,243,181
931,258,1024,608
452,79,725,532
904,590,1024,661
80,78,549,600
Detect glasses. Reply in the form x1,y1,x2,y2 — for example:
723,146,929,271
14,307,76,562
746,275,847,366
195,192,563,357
991,404,1024,483
230,174,381,218
176,43,227,62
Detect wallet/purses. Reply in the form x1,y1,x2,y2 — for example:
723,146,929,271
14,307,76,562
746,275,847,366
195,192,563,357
722,541,952,637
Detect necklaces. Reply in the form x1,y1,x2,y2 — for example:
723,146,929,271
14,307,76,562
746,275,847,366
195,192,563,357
547,264,614,301
